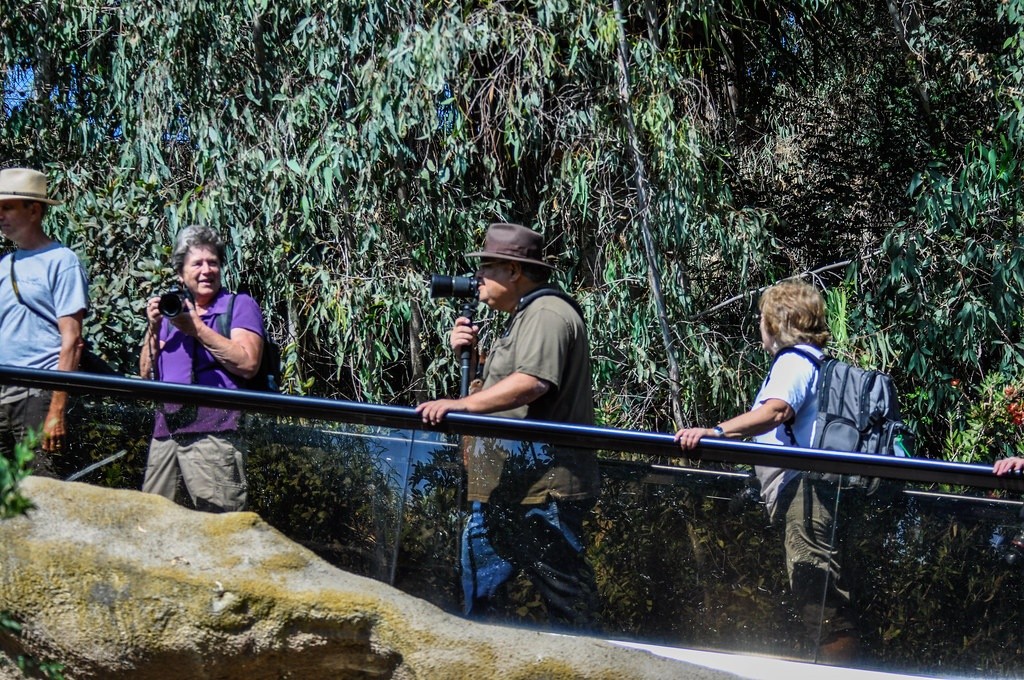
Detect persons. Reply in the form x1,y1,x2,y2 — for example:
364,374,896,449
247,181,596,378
415,222,608,636
0,168,90,476
140,225,265,512
992,456,1024,476
673,279,858,668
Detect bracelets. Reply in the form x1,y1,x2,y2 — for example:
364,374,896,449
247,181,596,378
714,425,724,438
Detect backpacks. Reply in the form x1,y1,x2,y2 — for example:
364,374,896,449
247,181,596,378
765,344,914,514
227,292,282,394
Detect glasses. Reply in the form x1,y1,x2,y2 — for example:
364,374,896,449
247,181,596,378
477,261,507,272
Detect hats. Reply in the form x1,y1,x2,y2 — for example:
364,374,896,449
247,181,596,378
464,223,562,273
0,168,61,205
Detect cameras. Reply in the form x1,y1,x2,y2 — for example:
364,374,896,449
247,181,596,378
157,278,194,317
430,272,482,299
